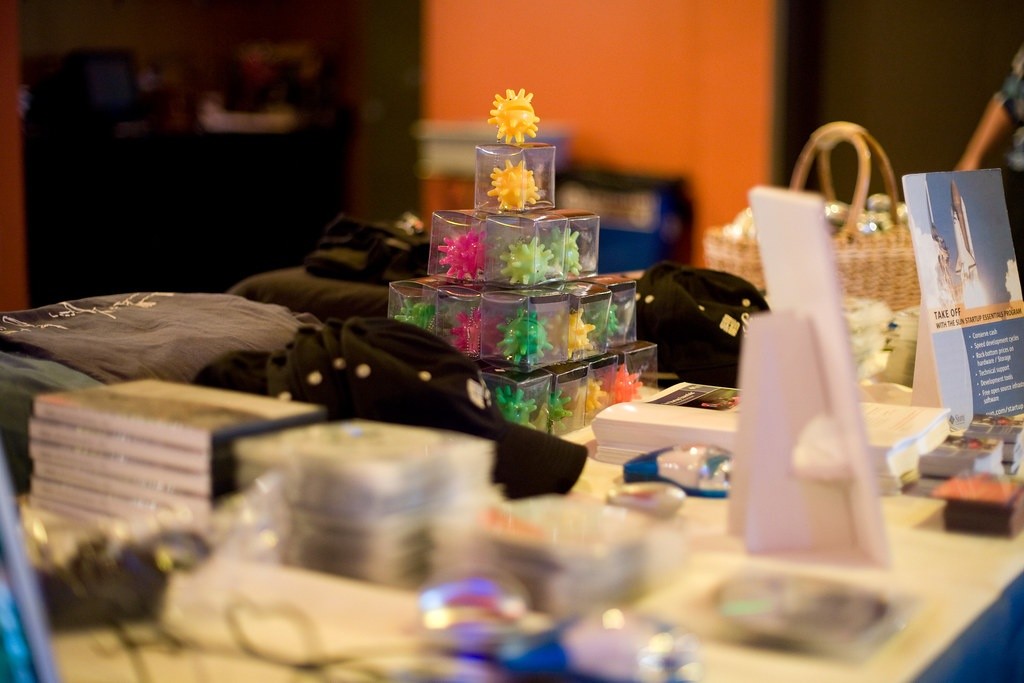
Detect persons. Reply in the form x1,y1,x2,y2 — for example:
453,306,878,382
950,44,1024,299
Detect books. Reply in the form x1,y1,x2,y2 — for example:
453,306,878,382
229,416,494,592
474,487,648,609
24,378,326,550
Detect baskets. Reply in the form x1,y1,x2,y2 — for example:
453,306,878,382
702,122,921,312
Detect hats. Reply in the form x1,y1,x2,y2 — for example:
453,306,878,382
192,316,589,501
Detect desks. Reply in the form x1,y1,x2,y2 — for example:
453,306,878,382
16,385,1024,683
